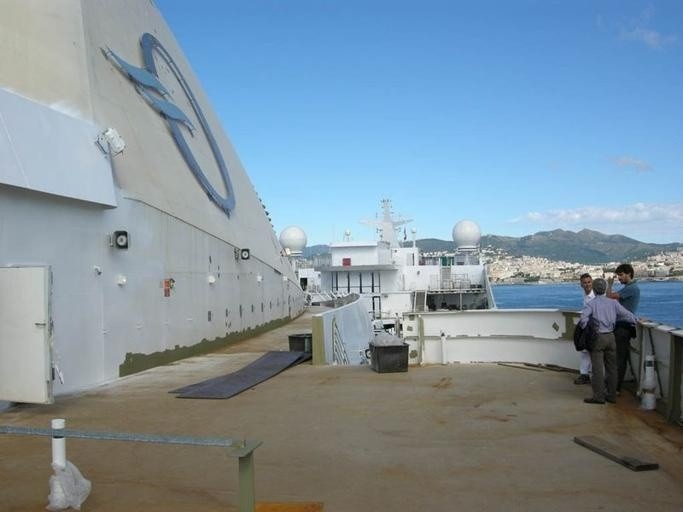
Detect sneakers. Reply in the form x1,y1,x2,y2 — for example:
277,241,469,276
574,374,591,384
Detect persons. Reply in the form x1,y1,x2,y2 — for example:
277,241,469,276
574,264,640,404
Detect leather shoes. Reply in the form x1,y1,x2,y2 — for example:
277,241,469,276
584,394,617,405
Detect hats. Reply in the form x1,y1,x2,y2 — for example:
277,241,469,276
592,279,607,294
